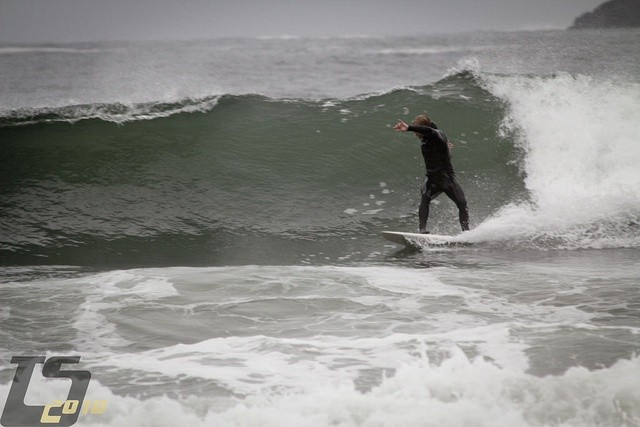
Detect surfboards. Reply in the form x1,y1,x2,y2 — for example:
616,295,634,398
380,231,459,245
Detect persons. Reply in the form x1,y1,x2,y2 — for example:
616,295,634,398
392,114,470,235
